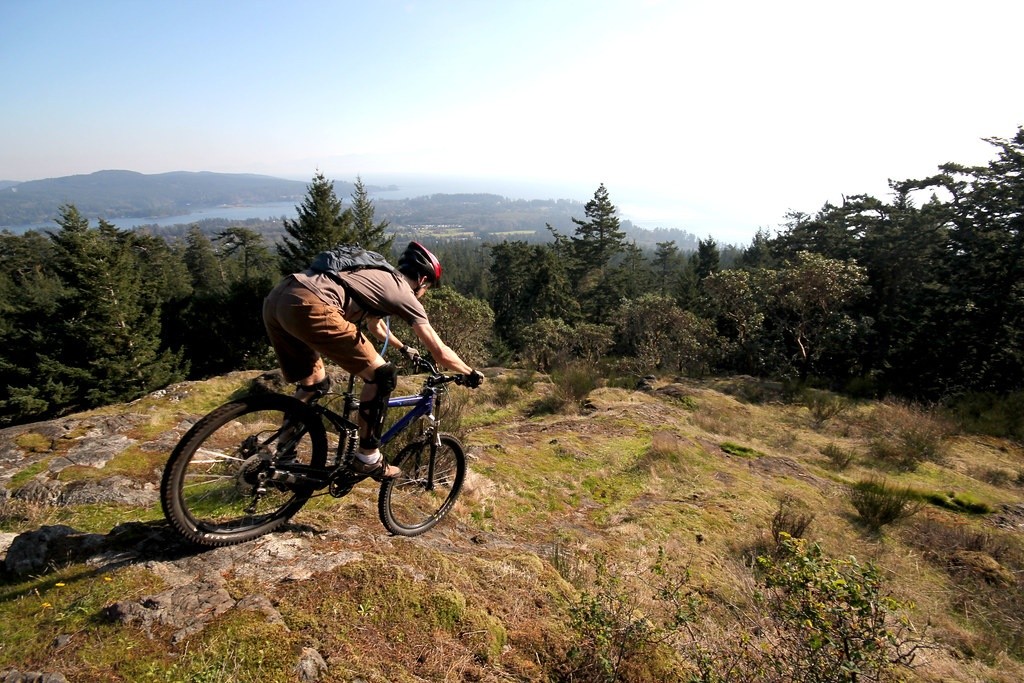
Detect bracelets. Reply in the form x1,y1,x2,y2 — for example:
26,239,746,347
400,345,408,353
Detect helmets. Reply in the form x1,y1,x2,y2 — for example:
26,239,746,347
398,241,441,280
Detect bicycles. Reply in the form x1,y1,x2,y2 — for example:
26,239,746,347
160,356,484,548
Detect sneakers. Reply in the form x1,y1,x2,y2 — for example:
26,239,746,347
352,456,402,479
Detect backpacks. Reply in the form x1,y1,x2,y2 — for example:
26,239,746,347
309,246,407,317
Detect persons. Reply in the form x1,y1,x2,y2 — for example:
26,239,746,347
263,242,483,478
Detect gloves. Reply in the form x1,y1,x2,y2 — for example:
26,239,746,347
466,370,484,389
399,346,420,361
